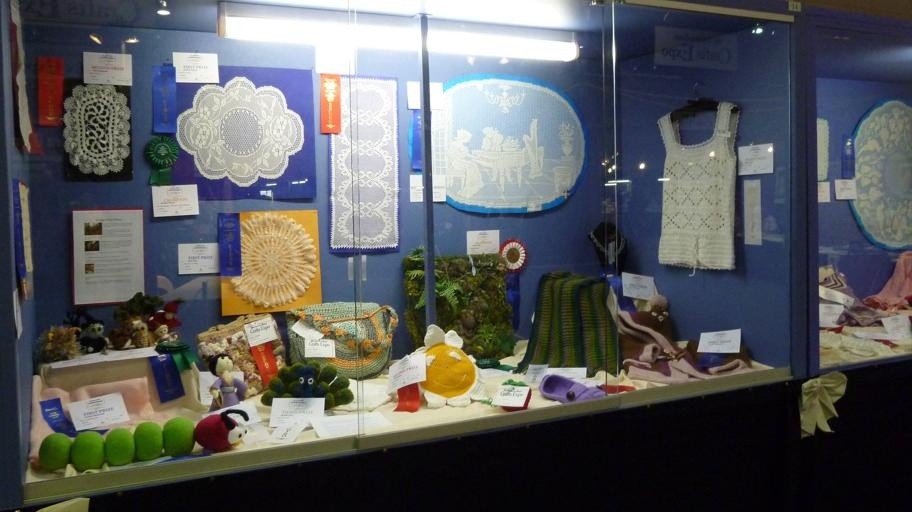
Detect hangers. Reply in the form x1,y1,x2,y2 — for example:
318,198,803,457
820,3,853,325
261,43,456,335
657,76,742,120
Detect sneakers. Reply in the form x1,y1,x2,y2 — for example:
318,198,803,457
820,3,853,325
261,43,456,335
539,375,608,404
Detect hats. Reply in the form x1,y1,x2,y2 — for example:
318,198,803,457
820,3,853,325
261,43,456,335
618,295,682,356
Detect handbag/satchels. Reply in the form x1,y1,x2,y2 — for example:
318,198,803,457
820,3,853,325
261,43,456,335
288,301,399,380
197,311,287,400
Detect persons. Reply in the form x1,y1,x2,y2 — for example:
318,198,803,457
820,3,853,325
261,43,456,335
208,353,250,409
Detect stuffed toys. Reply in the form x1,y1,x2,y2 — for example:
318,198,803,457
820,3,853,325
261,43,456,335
224,339,264,397
408,322,488,408
38,408,250,475
35,294,183,359
260,359,354,411
